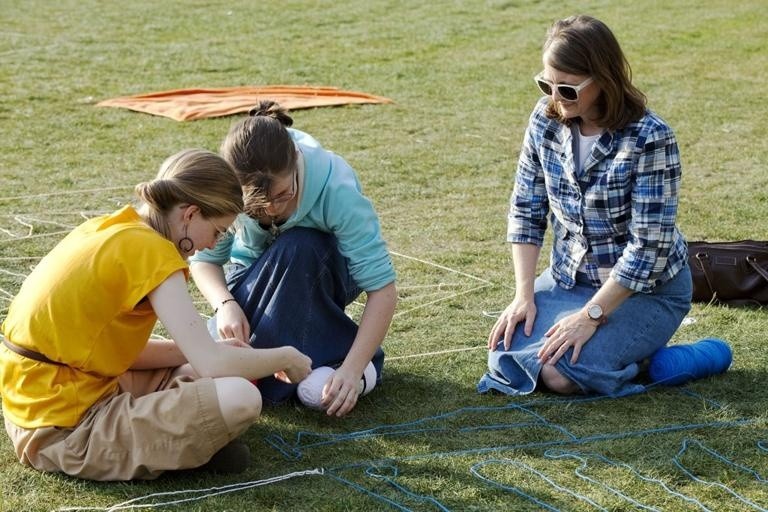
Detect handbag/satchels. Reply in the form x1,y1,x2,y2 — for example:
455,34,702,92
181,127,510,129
684,240,768,308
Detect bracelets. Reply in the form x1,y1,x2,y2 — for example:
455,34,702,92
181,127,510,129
213,296,236,316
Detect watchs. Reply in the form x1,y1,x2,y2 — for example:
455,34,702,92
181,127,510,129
582,302,607,328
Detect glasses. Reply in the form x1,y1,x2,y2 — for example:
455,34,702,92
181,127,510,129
268,170,294,202
534,69,593,102
180,204,227,241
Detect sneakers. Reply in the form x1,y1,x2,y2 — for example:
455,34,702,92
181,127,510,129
206,442,249,473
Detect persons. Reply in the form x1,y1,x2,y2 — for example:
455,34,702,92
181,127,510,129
477,15,738,399
184,98,401,417
0,148,314,480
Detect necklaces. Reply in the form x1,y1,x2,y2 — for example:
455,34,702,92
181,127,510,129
266,214,281,235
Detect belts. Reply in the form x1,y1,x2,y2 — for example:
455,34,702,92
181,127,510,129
4,339,69,369
